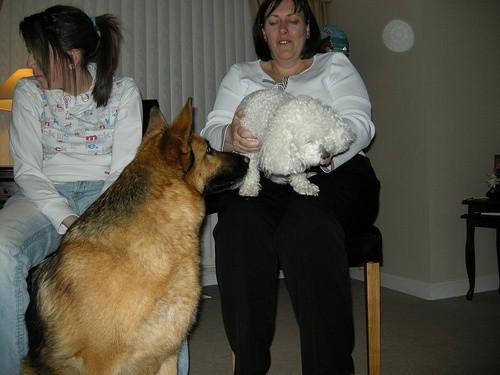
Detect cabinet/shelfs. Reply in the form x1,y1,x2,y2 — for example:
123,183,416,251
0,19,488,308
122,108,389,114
0,165,20,205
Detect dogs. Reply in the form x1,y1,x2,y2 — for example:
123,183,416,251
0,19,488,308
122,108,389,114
234,89,358,197
19,96,250,375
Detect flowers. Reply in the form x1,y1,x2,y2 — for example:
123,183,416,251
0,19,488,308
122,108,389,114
486,171,500,188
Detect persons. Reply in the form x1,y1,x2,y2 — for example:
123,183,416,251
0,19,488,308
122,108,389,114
0,6,143,375
200,0,376,375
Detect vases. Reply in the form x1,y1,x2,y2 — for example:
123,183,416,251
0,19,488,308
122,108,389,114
486,186,498,198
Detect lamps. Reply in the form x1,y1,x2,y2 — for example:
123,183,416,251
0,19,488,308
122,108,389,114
0,68,35,111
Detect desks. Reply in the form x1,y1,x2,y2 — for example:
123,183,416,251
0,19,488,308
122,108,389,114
461,198,500,300
